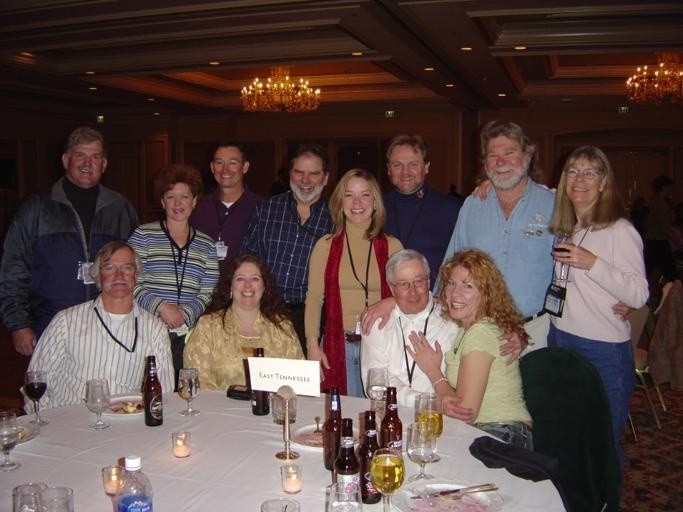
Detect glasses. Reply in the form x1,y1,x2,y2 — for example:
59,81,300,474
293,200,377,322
566,167,603,179
214,159,243,168
100,264,133,273
388,275,428,290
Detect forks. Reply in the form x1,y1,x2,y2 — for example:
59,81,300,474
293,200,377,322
446,487,498,500
314,416,323,438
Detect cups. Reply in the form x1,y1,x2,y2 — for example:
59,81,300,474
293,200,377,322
270,394,297,424
102,466,124,495
366,367,390,432
11,481,47,512
171,431,192,456
261,498,300,512
325,481,364,511
281,465,303,494
34,486,74,512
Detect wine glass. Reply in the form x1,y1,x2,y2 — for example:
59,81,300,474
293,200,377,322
370,448,404,511
406,422,438,482
0,412,19,472
414,394,444,463
550,229,574,284
83,378,111,432
21,370,50,427
176,368,202,416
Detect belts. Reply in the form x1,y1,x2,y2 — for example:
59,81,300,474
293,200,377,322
524,310,546,323
345,334,361,341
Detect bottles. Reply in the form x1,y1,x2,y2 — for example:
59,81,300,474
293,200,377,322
360,410,380,504
334,418,359,501
143,354,163,427
321,387,342,471
251,347,269,414
225,384,256,402
379,386,403,455
115,455,153,512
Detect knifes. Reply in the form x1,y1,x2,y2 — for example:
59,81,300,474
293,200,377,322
409,483,495,499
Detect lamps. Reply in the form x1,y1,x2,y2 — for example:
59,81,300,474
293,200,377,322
623,50,682,107
239,65,321,113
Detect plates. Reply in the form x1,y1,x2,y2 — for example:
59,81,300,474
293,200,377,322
288,421,359,450
390,478,502,511
101,392,167,416
0,424,40,449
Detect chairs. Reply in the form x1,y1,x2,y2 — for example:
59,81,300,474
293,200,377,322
518,348,619,511
626,304,666,444
